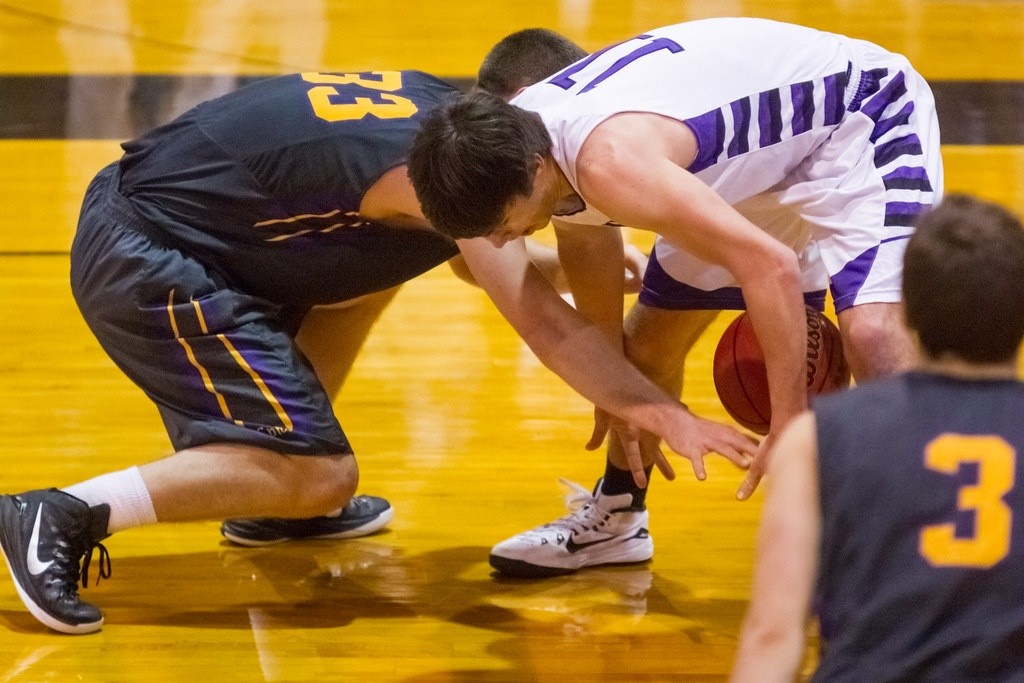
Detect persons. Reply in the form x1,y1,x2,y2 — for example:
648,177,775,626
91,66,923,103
730,194,1024,683
0,25,762,636
408,17,945,577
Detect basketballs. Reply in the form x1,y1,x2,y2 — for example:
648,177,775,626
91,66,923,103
714,303,852,438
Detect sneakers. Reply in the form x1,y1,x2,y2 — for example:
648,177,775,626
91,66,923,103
0,487,113,634
220,493,393,547
489,477,654,576
